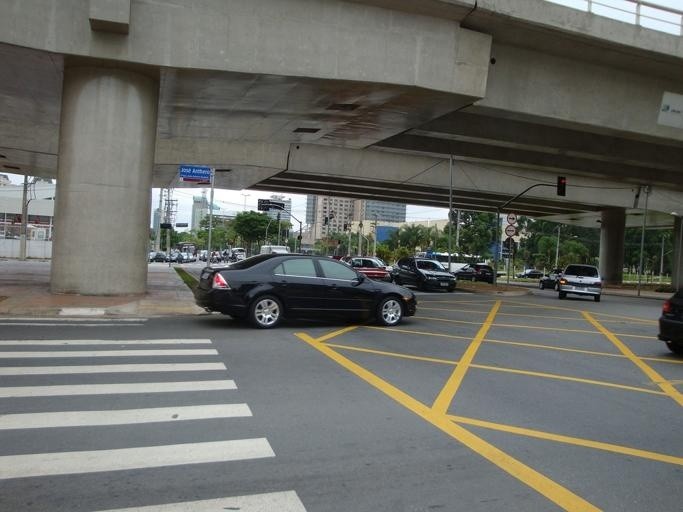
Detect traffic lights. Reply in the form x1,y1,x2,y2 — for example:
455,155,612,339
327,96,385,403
556,176,566,196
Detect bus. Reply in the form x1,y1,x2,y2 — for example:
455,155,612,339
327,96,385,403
414,250,490,275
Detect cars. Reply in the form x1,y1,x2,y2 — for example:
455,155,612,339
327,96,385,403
538,269,568,292
454,263,497,284
389,255,458,292
656,285,683,359
146,241,295,266
515,269,545,280
557,263,605,303
191,252,419,329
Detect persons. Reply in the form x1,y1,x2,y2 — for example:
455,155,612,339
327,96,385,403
212,250,237,263
